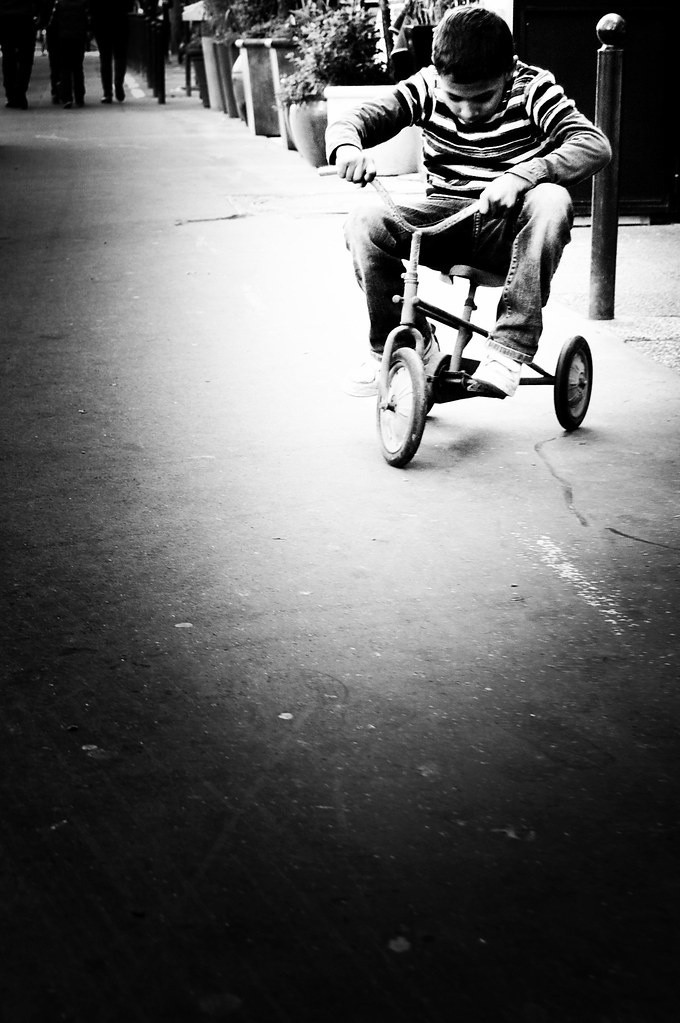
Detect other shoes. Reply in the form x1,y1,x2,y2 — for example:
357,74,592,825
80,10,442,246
101,96,112,104
115,84,125,102
5,95,29,110
64,95,84,109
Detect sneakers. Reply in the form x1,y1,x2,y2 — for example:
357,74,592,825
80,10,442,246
339,351,389,397
471,346,521,397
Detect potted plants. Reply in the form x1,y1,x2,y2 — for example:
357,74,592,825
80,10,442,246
271,35,329,168
302,6,420,175
271,4,325,150
235,19,281,136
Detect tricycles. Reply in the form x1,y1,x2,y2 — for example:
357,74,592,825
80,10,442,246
313,162,596,470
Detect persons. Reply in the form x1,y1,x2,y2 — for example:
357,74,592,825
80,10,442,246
0,0,130,109
325,7,612,396
